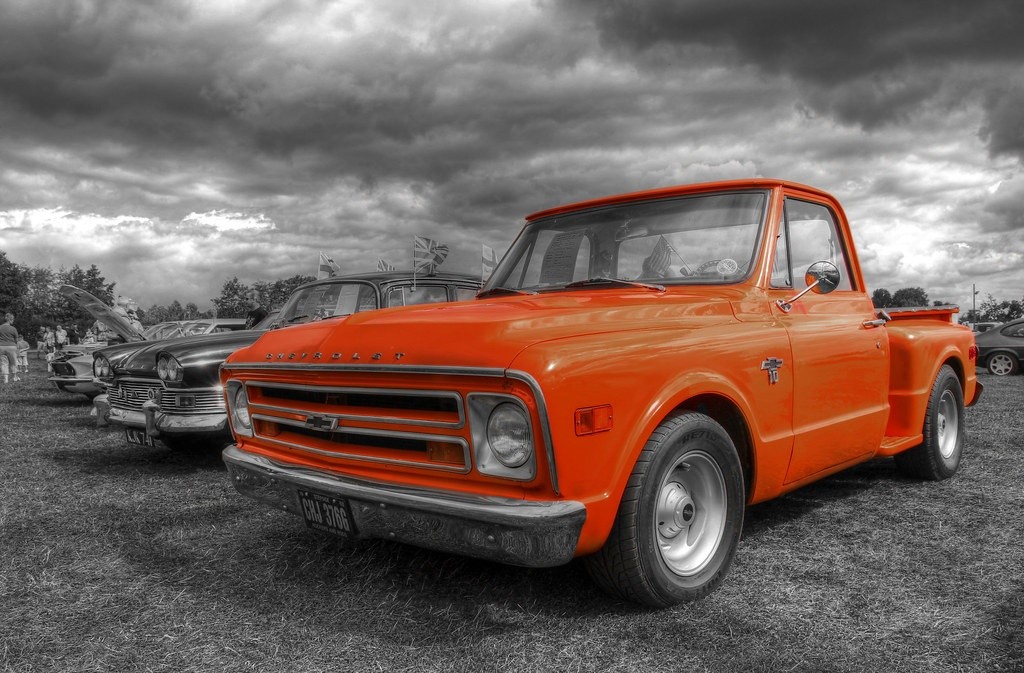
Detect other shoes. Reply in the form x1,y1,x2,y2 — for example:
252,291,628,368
4,380,8,384
17,370,22,373
13,377,20,381
25,370,28,373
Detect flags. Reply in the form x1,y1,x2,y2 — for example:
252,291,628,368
414,236,449,273
318,253,338,280
646,237,674,276
376,260,396,272
482,245,500,281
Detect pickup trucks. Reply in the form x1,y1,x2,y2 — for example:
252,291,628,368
230,180,984,608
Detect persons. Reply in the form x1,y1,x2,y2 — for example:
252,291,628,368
0,313,20,383
113,297,143,332
36,323,86,359
16,336,30,373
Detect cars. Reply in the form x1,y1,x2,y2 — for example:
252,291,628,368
89,272,486,456
48,284,247,400
974,317,1024,376
